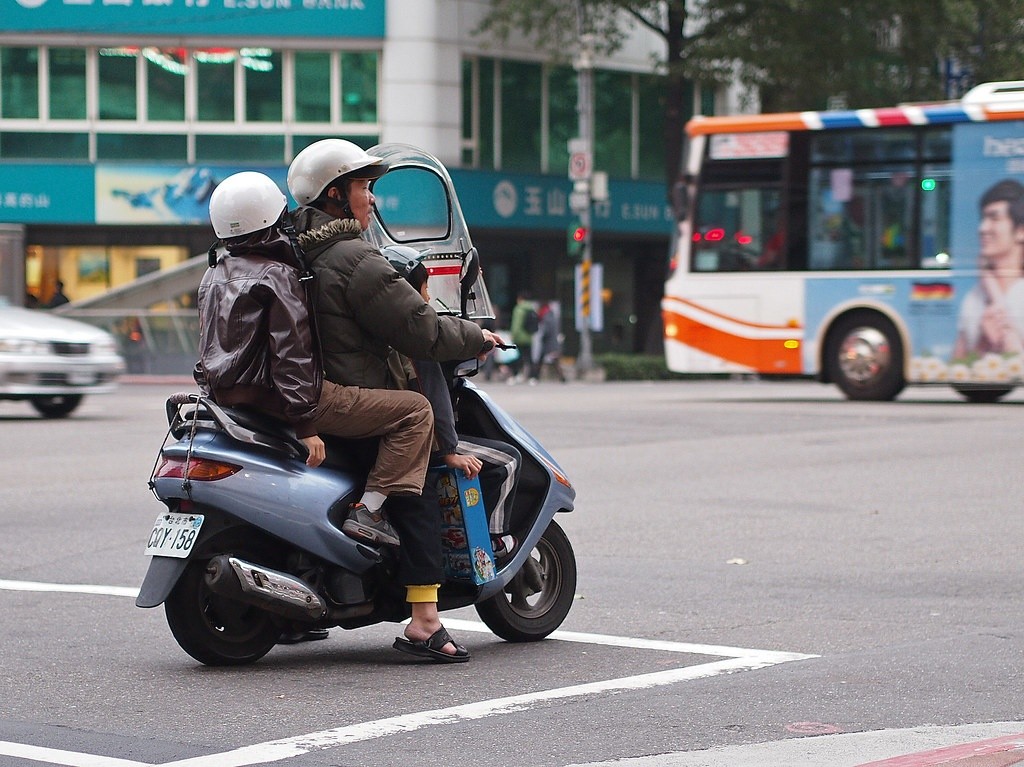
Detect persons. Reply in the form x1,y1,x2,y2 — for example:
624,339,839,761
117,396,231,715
48,281,69,308
199,138,503,661
756,204,786,271
953,180,1024,358
487,291,567,384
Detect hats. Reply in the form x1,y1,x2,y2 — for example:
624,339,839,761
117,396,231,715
342,164,388,180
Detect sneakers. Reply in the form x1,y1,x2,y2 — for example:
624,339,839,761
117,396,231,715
341,503,400,547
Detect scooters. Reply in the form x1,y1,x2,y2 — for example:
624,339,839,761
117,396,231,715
135,145,578,667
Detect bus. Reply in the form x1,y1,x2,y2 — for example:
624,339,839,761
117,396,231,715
661,80,1024,400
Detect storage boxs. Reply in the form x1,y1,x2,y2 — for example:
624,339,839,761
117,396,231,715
432,461,498,587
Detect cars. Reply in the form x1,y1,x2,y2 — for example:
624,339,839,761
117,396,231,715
0,298,124,417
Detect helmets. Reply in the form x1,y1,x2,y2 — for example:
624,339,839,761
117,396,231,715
208,171,286,239
379,245,431,286
286,138,384,208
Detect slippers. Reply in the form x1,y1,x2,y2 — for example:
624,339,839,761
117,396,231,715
392,623,470,663
